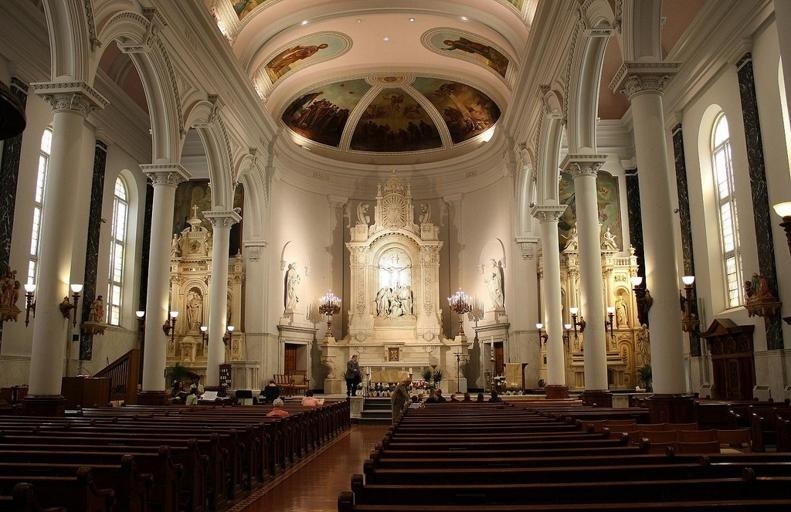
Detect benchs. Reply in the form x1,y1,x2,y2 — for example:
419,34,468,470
341,398,790,512
1,402,352,512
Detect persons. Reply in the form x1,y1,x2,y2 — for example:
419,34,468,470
285,262,301,310
167,375,325,418
480,258,504,309
561,222,579,250
603,226,620,249
615,295,628,325
345,355,503,425
744,273,773,298
440,40,502,66
187,293,202,330
271,43,328,74
90,295,104,321
0,272,20,304
282,91,349,138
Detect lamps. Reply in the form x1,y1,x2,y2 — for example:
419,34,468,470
135,309,179,342
200,325,235,349
773,200,791,245
631,275,695,319
23,283,82,327
569,306,615,340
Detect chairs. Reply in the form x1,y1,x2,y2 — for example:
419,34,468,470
272,372,310,397
